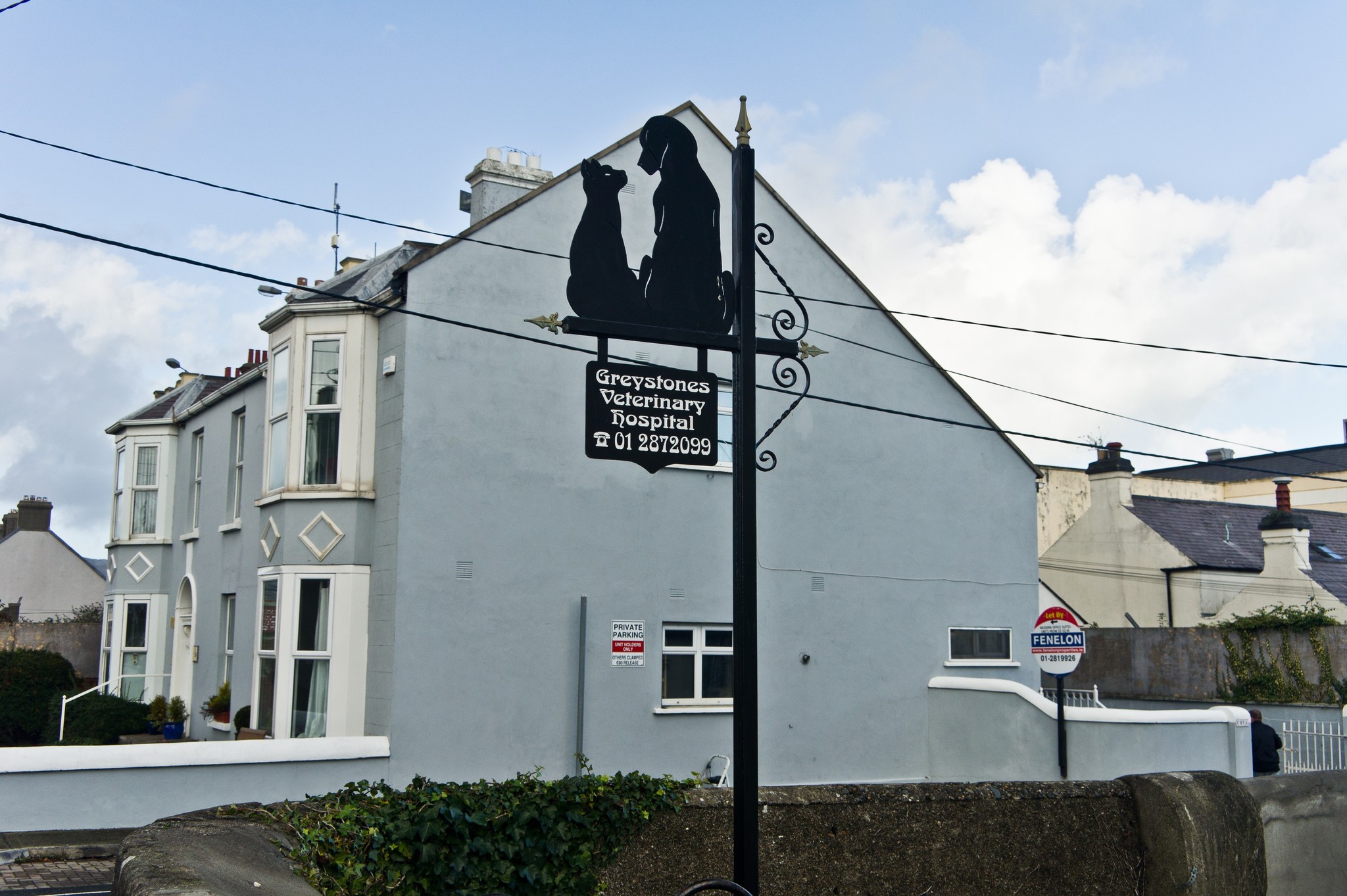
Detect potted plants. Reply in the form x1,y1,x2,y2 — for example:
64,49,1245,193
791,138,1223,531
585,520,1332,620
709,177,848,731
199,679,231,725
146,693,168,736
161,695,189,741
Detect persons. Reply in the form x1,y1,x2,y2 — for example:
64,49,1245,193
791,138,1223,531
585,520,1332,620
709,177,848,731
1248,709,1282,777
